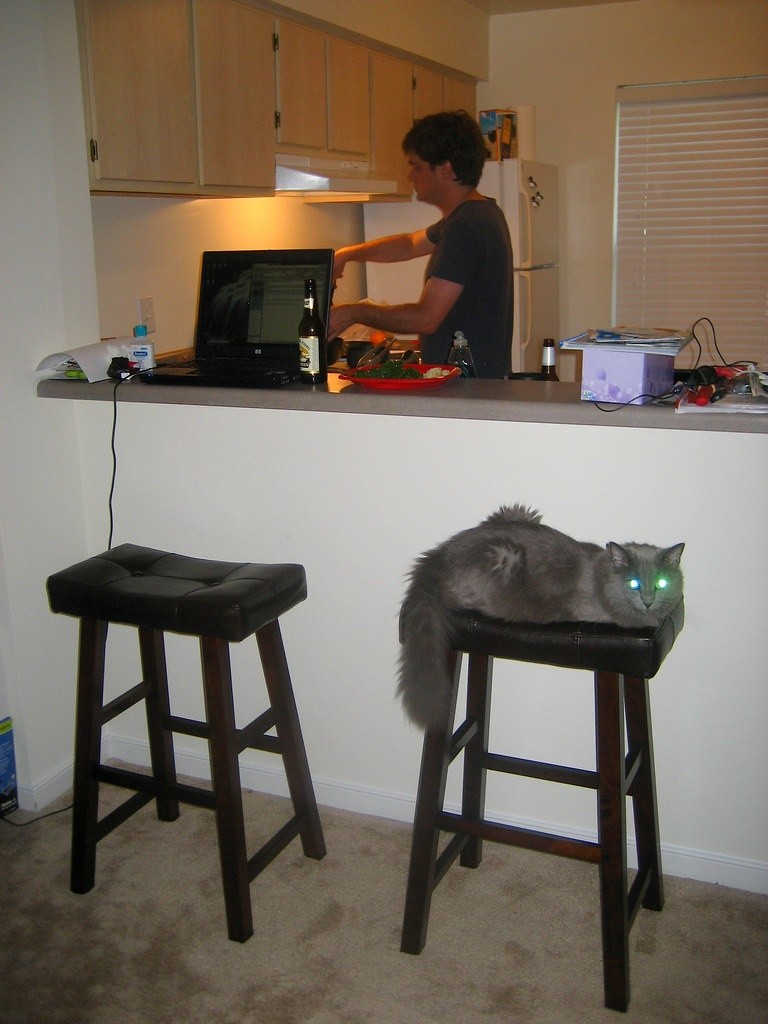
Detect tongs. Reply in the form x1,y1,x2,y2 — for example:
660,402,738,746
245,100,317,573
356,336,397,366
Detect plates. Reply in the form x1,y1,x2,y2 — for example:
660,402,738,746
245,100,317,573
337,363,462,389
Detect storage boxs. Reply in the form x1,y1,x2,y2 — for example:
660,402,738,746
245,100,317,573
477,109,519,160
0,713,18,819
581,348,675,405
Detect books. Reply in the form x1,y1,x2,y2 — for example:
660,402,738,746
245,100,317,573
559,325,694,357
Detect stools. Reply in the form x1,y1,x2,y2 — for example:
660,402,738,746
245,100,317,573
46,543,328,943
394,595,685,1012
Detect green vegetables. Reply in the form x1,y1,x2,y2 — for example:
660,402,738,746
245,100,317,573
353,360,424,379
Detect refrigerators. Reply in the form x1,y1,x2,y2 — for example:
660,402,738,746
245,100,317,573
363,158,559,377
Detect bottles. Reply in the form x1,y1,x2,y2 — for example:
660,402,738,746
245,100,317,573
130,326,155,371
536,339,559,382
298,278,326,385
447,331,477,379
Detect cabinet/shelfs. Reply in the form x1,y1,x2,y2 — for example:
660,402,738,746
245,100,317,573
76,0,492,204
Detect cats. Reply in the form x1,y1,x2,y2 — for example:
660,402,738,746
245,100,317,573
394,504,685,732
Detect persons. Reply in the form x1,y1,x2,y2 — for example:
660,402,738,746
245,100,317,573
326,110,513,380
